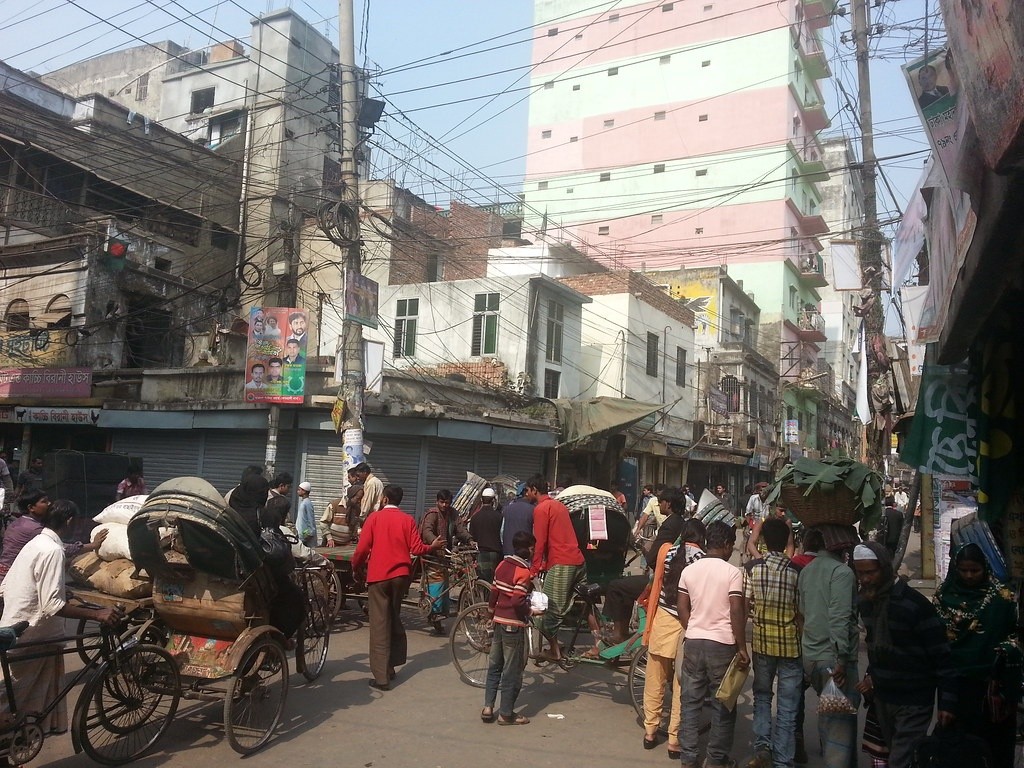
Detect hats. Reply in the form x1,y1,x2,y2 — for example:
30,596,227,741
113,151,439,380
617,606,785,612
482,487,495,497
299,481,311,492
852,545,878,560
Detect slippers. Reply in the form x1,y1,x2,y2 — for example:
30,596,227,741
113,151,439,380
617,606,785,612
602,635,622,648
667,746,680,760
581,649,604,660
498,712,530,725
528,649,559,665
644,733,658,749
481,707,494,720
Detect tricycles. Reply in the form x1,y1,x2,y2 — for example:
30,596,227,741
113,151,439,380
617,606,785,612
450,584,712,737
76,535,341,753
0,593,181,768
311,540,492,652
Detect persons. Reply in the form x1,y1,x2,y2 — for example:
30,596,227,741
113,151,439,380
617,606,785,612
643,518,706,759
798,526,859,768
0,455,154,556
267,358,284,384
744,518,805,768
931,543,1024,768
0,498,124,768
677,521,751,768
246,363,271,388
281,339,305,365
481,530,545,725
351,483,448,691
854,542,943,768
0,487,109,615
253,309,282,337
287,311,308,345
225,465,922,664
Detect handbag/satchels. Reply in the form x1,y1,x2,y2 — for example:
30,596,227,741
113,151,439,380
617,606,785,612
815,668,858,716
911,721,992,768
256,506,299,573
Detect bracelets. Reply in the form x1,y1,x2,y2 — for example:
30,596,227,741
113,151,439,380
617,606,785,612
838,662,847,668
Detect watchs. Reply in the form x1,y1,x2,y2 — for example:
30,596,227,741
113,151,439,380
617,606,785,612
74,540,86,554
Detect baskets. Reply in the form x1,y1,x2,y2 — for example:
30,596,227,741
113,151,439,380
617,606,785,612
780,483,871,527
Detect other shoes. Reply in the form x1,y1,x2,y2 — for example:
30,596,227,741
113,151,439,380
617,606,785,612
389,669,396,680
757,748,774,768
794,750,809,764
369,678,388,691
430,627,446,636
